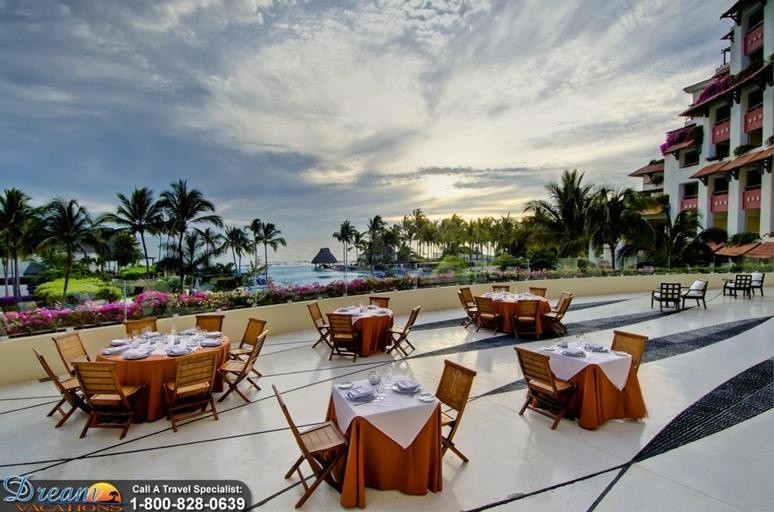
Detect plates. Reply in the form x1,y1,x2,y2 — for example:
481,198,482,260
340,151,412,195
336,384,354,389
417,395,435,402
390,385,424,394
562,352,585,357
109,344,127,347
201,343,221,346
166,348,193,355
344,395,376,402
122,352,152,359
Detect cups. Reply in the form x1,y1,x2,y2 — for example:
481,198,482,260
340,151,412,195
132,330,139,346
124,334,131,346
146,325,152,339
141,328,148,343
368,370,379,396
381,365,393,390
171,323,177,335
575,335,583,350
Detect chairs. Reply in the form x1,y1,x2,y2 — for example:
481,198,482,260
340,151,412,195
226,317,267,376
549,291,572,329
456,290,478,328
271,384,347,509
611,330,648,374
492,286,510,292
743,273,765,297
529,287,547,298
218,329,269,402
651,283,681,312
724,275,752,299
306,302,334,348
674,279,708,310
123,317,157,337
196,315,226,332
368,296,390,308
71,362,145,439
459,287,476,306
512,300,542,340
542,296,574,337
474,296,502,335
326,314,358,362
436,359,476,462
33,348,89,428
163,351,219,432
513,346,575,430
385,305,422,356
52,332,91,377
